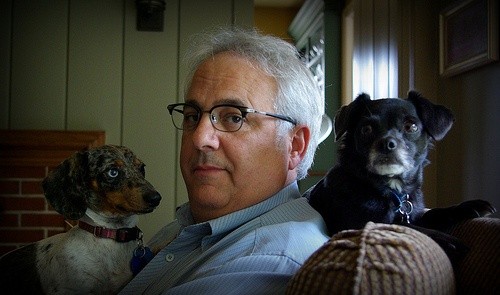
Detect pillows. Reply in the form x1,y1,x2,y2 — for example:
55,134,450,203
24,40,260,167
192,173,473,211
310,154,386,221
286,220,455,295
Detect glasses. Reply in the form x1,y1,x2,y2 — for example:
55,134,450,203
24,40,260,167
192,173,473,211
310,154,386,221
167,102,298,132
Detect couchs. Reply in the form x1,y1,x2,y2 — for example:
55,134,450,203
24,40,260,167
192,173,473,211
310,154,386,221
421,208,500,295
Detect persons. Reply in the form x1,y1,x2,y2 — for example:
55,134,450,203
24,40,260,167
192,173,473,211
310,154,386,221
109,22,334,295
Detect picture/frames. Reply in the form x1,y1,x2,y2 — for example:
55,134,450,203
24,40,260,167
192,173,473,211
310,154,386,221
438,0,499,79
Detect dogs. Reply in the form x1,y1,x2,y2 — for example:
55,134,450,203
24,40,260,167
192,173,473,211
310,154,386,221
301,92,497,257
0,144,162,295
